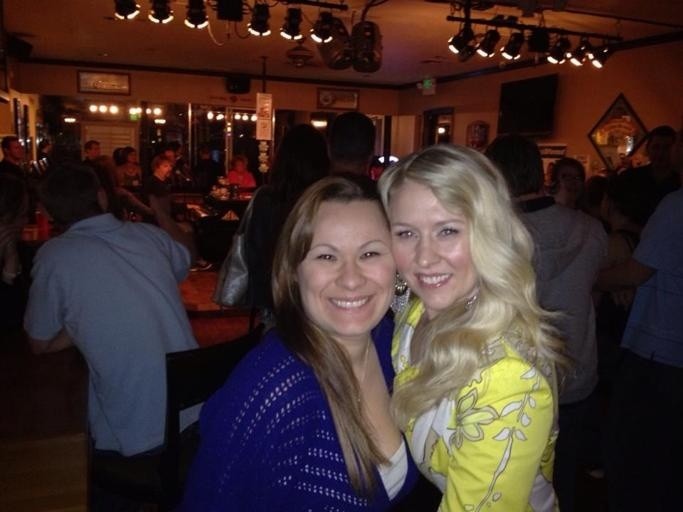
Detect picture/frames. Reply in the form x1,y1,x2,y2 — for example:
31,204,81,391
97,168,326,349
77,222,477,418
583,89,652,174
315,86,361,112
75,68,132,96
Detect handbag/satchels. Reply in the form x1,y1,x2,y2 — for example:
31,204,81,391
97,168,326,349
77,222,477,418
209,183,264,307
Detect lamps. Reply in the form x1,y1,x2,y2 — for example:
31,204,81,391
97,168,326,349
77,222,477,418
146,0,174,26
565,32,592,67
446,22,473,56
473,23,501,59
183,0,211,30
309,3,335,43
586,37,618,69
114,0,141,21
245,2,272,38
547,29,568,65
497,26,526,60
278,6,306,41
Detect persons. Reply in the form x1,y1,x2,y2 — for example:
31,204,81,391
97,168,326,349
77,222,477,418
190,143,221,190
213,123,331,307
148,152,213,272
593,181,642,339
544,158,591,210
222,153,254,190
484,131,612,406
173,141,194,173
0,174,37,353
594,129,681,512
164,147,185,190
182,174,443,511
23,165,217,510
83,140,154,223
37,138,51,160
321,111,382,196
378,143,560,512
1,135,25,174
609,125,681,246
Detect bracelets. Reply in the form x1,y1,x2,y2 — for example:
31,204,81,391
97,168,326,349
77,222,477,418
2,266,21,282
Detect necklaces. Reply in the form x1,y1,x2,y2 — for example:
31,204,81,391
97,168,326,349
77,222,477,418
324,342,369,406
